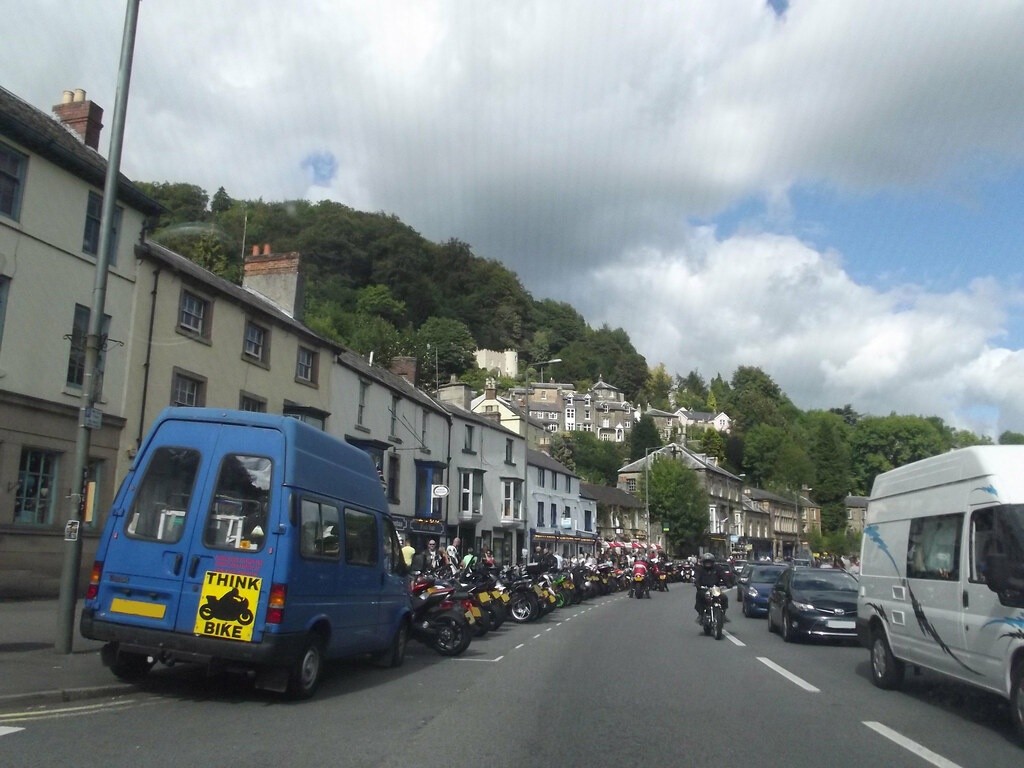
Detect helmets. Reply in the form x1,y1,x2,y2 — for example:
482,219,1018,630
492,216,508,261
701,552,715,568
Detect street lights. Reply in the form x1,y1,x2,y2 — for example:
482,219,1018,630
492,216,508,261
523,359,563,563
795,488,813,555
645,445,682,546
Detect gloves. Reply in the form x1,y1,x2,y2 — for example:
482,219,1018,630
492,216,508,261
727,583,732,589
696,585,701,590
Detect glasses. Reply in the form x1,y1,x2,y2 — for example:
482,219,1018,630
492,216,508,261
429,544,435,545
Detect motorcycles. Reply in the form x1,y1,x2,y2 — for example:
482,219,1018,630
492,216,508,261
699,585,732,641
406,545,696,656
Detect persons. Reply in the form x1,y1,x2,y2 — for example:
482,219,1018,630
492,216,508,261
383,531,671,600
759,554,792,563
694,552,737,623
688,554,697,565
726,554,734,563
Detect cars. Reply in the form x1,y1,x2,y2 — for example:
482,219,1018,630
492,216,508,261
794,557,814,566
737,563,791,618
767,565,863,644
718,559,748,584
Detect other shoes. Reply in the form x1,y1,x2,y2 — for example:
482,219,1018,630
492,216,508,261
725,617,730,622
697,615,703,622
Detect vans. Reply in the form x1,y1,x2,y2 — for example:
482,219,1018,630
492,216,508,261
80,403,416,701
856,443,1024,745
